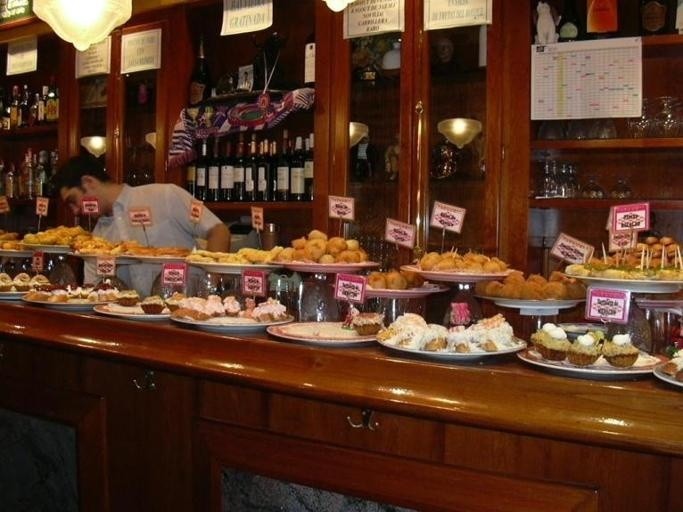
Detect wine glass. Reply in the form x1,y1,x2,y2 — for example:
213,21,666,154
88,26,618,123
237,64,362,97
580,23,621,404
626,98,654,138
536,117,618,140
654,95,679,137
541,160,578,198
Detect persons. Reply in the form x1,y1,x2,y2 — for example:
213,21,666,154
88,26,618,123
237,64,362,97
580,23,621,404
49,154,231,304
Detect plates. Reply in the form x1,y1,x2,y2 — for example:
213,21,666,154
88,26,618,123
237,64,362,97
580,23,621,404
363,284,449,301
399,262,520,283
68,250,137,264
374,332,528,362
120,253,186,265
0,290,27,301
21,283,116,311
471,293,586,308
265,320,386,346
92,296,171,323
187,261,281,274
0,249,31,257
514,344,658,379
564,261,683,294
266,260,379,273
652,358,683,385
18,244,70,253
168,300,294,333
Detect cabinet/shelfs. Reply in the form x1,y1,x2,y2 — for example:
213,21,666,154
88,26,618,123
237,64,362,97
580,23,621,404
329,0,497,277
167,2,327,256
499,0,683,303
68,3,165,232
0,15,67,241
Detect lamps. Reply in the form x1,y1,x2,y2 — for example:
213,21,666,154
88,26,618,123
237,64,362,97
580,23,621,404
436,115,485,150
77,133,108,158
350,121,368,148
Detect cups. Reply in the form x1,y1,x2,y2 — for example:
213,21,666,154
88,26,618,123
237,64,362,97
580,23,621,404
645,308,669,353
272,275,294,312
608,176,632,199
580,175,605,199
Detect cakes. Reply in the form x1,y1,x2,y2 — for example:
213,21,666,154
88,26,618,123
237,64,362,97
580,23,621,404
166,292,187,313
116,289,140,306
352,312,384,335
366,269,424,290
536,326,570,361
23,290,51,302
141,294,166,314
567,333,602,367
661,347,683,383
0,272,13,292
565,261,683,281
603,334,641,368
67,283,119,304
530,322,557,352
31,275,50,290
51,289,67,303
269,230,369,264
14,273,31,291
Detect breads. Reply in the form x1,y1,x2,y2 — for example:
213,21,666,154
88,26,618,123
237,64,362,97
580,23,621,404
0,226,191,264
416,246,509,275
474,271,585,301
589,236,683,267
377,312,515,353
179,295,288,323
184,247,269,264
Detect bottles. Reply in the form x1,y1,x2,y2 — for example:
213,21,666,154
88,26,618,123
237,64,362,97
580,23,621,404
234,130,246,202
0,147,59,199
637,0,677,36
436,27,455,81
432,138,474,182
185,139,196,198
304,137,311,161
188,28,215,108
196,136,211,203
0,255,56,280
207,138,219,203
382,122,400,184
278,126,290,202
290,135,305,202
219,135,235,202
258,138,277,202
305,23,315,88
536,159,575,199
306,133,314,202
0,76,60,131
350,124,375,184
247,132,259,202
